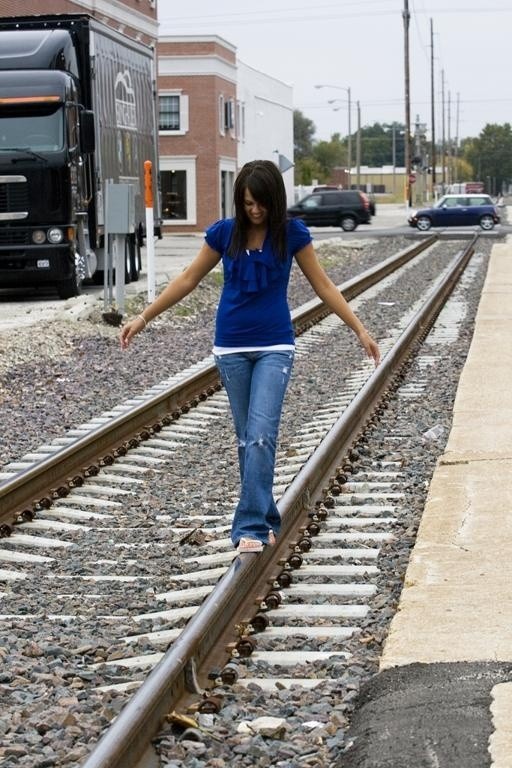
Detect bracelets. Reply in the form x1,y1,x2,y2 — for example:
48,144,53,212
139,315,147,330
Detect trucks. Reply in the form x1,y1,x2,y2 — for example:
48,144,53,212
1,13,162,294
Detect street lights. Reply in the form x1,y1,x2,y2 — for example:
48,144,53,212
314,83,362,185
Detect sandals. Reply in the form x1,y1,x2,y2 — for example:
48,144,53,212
236,529,276,554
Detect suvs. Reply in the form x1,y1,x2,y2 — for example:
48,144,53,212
288,189,375,232
406,194,501,230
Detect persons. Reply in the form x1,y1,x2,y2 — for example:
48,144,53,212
455,198,464,207
119,161,382,555
